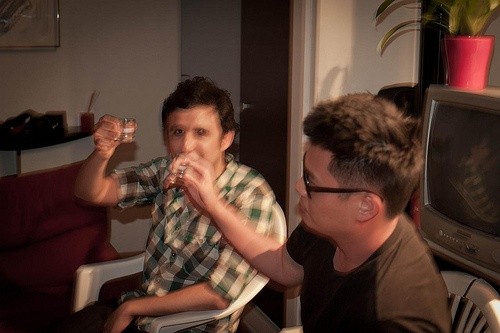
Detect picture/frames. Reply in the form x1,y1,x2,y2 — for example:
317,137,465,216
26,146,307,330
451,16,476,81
0,0,60,53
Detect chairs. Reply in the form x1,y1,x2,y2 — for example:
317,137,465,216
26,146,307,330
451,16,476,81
440,271,500,333
71,200,287,333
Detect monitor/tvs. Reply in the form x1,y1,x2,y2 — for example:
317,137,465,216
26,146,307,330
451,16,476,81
418,85,500,276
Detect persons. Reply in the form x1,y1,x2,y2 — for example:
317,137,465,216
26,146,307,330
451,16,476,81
39,76,288,333
455,136,498,234
163,92,452,333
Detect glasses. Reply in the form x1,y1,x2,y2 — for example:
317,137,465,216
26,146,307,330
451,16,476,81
302,151,384,203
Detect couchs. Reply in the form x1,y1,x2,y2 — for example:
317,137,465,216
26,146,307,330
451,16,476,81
0,160,141,333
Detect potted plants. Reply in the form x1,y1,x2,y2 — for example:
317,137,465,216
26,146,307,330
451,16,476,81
375,0,500,91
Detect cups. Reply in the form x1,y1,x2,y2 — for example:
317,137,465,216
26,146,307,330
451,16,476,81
80,113,94,132
175,157,188,182
118,118,137,142
